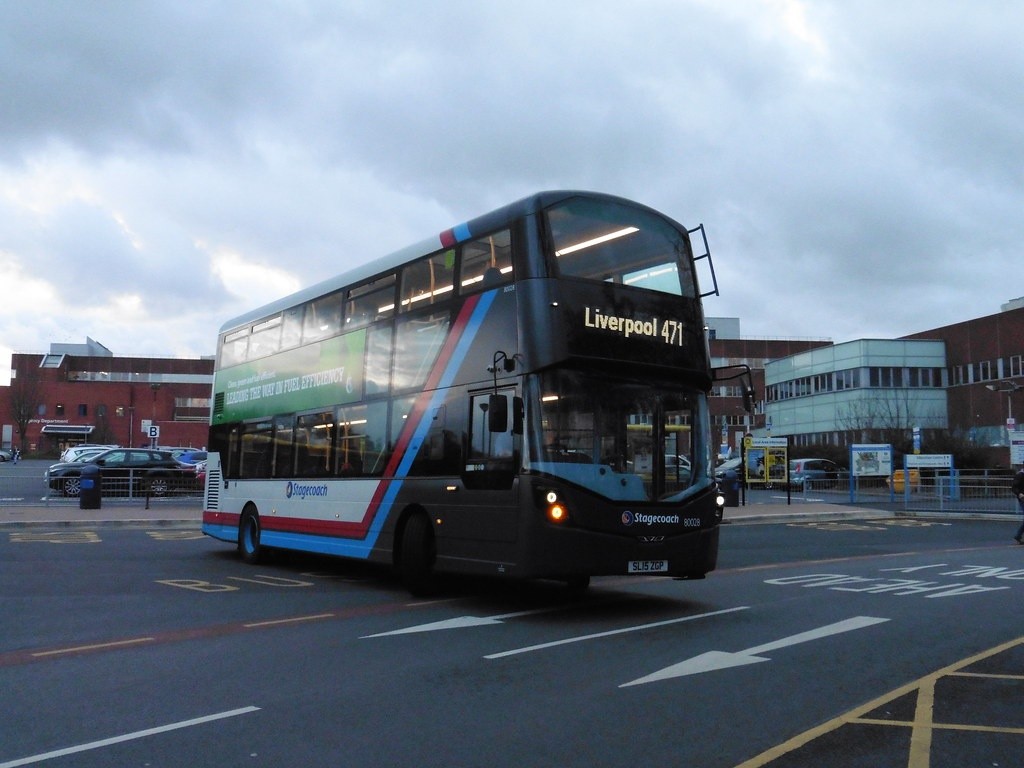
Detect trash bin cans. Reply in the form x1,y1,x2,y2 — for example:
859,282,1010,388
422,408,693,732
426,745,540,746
886,469,920,495
721,469,739,507
80,464,102,509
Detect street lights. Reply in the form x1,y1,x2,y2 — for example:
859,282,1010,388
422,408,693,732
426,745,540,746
985,385,1015,431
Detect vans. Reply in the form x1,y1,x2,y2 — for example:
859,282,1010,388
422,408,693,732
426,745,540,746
789,459,846,489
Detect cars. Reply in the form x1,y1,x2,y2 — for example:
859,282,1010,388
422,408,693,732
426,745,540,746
0,450,10,461
715,455,744,480
662,454,690,477
157,446,207,490
59,443,123,465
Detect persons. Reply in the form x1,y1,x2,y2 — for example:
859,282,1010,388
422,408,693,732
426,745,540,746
1011,460,1024,545
12,445,20,464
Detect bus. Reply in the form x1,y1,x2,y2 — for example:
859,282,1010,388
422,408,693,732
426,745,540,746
201,191,756,605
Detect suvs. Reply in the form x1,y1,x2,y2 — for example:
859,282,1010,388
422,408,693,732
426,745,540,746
45,448,182,496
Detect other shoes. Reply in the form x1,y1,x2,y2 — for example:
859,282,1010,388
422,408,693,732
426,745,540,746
1015,534,1024,544
